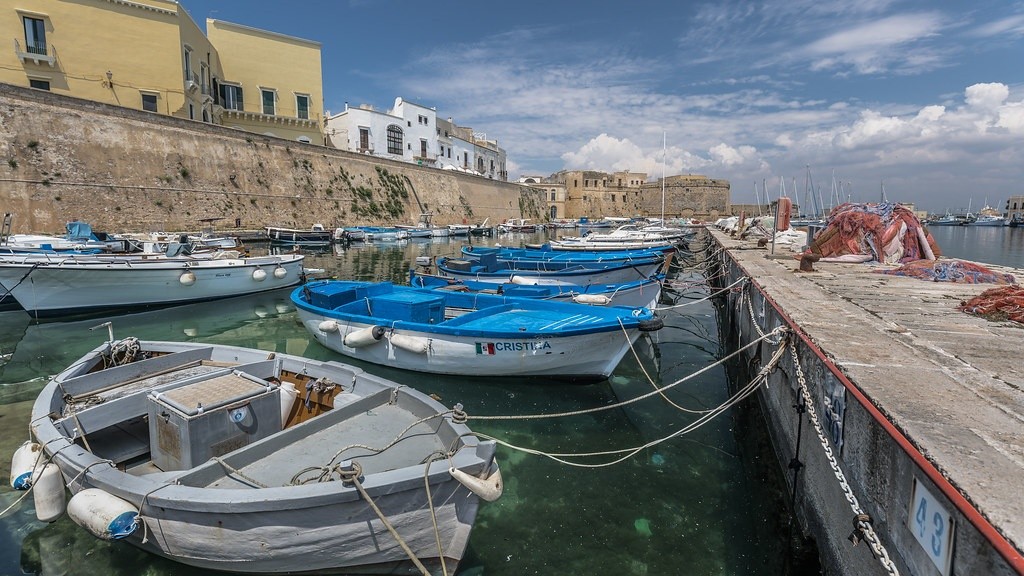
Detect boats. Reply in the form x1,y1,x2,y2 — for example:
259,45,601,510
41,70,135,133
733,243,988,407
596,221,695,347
8,336,503,576
0,132,698,322
0,248,309,325
288,279,664,384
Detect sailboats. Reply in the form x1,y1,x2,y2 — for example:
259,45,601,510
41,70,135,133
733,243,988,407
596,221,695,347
713,162,1024,236
